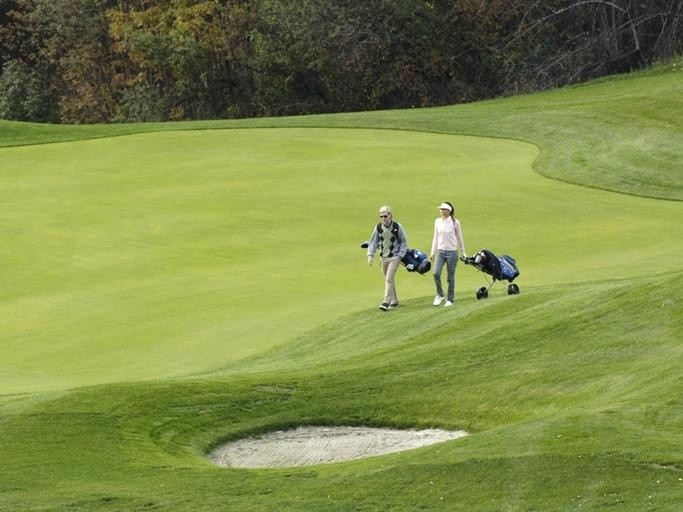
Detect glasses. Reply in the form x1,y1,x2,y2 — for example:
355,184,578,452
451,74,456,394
380,215,388,218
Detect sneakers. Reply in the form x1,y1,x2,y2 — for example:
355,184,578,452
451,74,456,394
379,305,387,311
444,301,454,306
433,296,445,305
389,303,399,309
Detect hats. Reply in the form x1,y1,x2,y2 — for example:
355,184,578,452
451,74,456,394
438,203,452,211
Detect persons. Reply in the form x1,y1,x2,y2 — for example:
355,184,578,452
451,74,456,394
368,206,409,310
430,201,468,307
